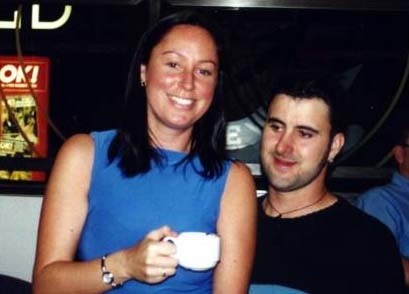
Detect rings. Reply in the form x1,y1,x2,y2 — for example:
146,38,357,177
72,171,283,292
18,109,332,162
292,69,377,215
162,272,168,277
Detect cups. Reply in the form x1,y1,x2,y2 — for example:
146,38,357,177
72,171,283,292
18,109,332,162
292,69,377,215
162,232,221,272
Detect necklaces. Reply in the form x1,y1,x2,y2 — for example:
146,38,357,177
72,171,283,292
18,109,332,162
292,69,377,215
267,192,327,221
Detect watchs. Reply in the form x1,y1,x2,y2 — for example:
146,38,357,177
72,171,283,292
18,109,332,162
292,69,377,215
101,254,122,289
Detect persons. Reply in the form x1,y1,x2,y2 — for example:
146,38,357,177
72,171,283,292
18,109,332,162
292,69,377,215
246,80,406,294
32,10,258,294
353,129,409,287
